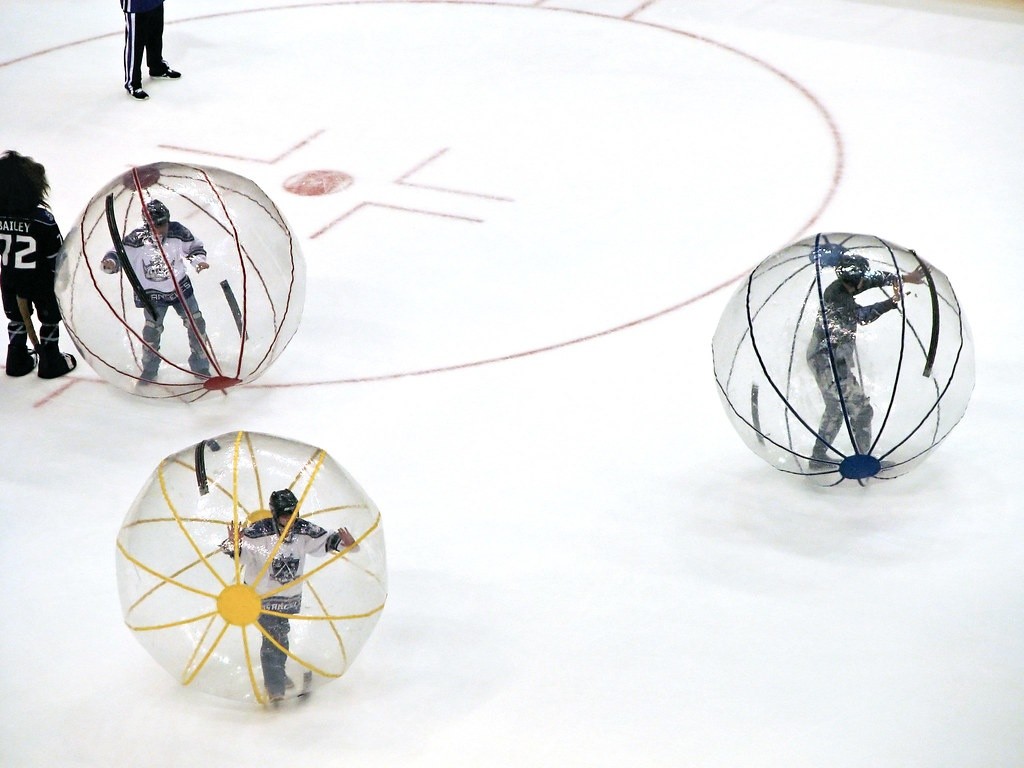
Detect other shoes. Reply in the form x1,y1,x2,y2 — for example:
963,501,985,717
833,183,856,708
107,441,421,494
880,461,895,471
194,371,211,384
280,674,294,690
809,457,841,471
269,693,286,706
139,368,158,385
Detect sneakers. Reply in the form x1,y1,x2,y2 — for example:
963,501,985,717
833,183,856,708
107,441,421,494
151,68,182,79
133,89,150,101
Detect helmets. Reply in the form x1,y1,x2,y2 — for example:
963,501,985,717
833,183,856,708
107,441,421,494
141,200,170,228
835,254,869,286
270,490,298,521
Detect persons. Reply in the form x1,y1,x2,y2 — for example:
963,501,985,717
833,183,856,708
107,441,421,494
806,255,934,471
0,151,77,380
99,198,212,388
119,0,182,101
217,489,359,712
